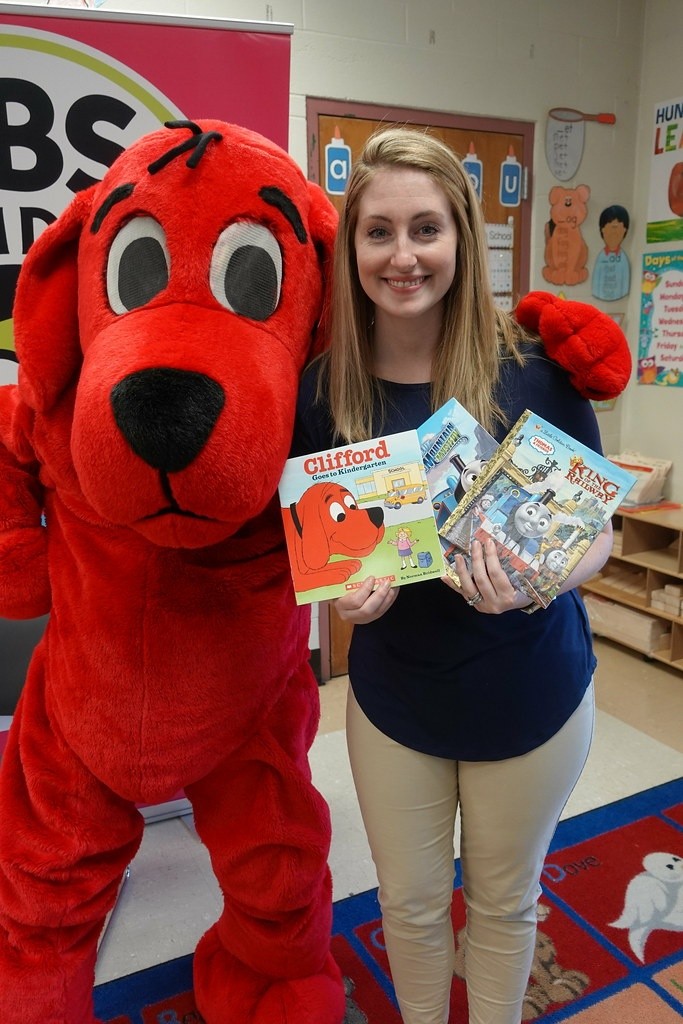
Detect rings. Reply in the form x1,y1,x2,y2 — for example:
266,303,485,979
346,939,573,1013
468,592,483,608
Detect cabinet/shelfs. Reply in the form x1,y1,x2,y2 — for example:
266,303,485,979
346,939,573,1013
578,500,683,671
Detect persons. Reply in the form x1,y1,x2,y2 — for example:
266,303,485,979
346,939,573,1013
286,128,615,1024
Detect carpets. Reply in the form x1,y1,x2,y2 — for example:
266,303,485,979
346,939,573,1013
92,776,683,1024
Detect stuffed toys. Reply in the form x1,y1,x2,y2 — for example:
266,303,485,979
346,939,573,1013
0,115,631,1024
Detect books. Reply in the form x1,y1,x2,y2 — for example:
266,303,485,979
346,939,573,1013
279,395,637,610
